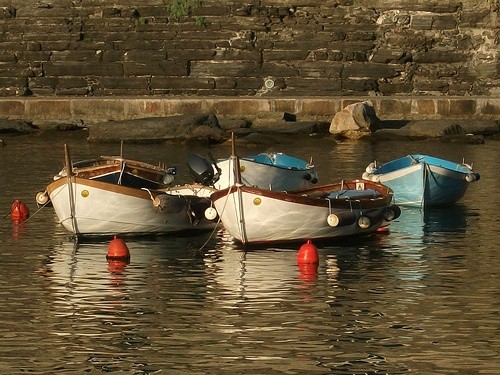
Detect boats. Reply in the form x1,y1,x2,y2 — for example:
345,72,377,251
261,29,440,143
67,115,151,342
212,132,314,192
72,140,166,190
46,143,220,238
362,154,474,208
210,155,394,246
